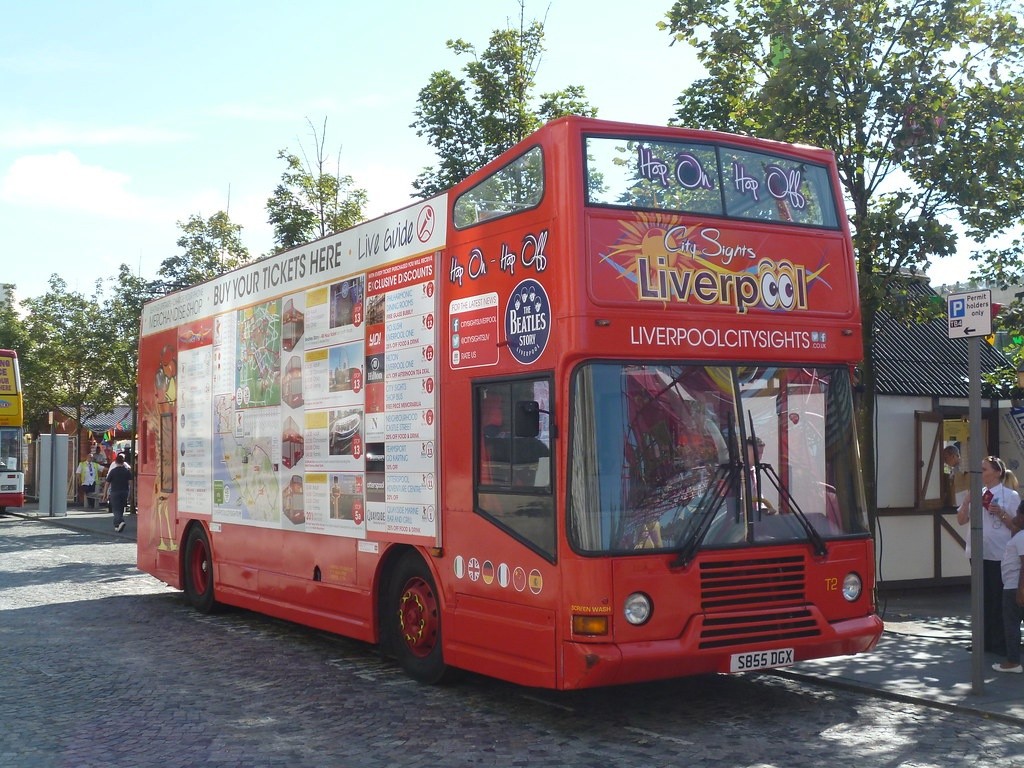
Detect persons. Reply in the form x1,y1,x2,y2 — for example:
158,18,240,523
102,454,134,532
957,456,1024,673
740,436,766,511
943,445,971,508
76,444,132,509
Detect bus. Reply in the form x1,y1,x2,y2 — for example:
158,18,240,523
0,348,32,515
138,115,885,689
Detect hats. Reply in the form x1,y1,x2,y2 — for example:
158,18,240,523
124,444,131,449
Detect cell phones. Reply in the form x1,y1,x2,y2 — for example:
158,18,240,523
990,497,999,515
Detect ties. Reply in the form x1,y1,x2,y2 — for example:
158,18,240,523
89,462,93,476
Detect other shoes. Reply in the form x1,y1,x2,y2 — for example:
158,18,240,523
992,663,1024,673
965,644,973,652
115,521,126,532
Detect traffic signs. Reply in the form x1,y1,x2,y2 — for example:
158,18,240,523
948,290,992,339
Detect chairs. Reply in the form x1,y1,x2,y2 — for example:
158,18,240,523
488,435,551,526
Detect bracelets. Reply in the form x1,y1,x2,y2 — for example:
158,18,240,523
1000,512,1007,522
